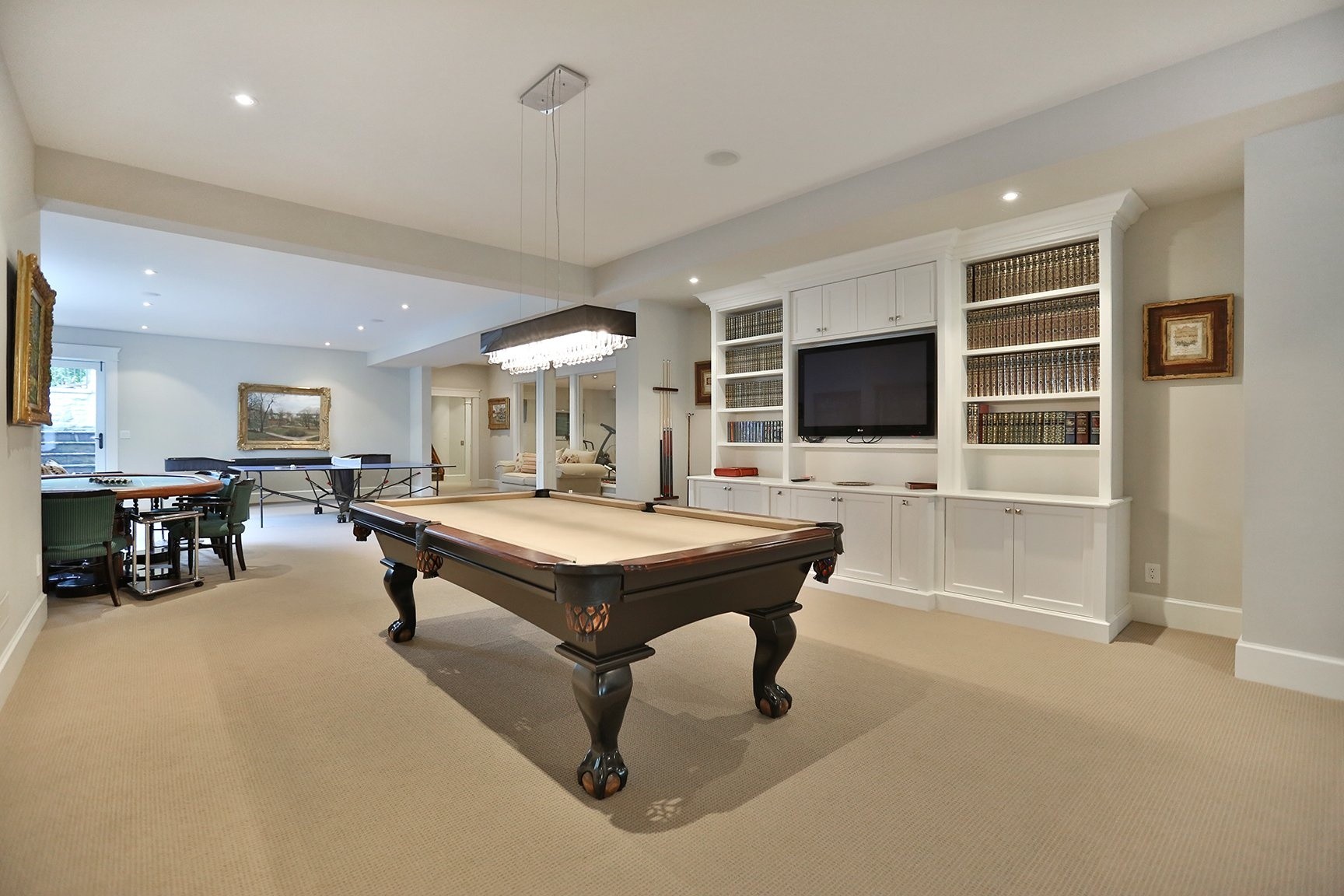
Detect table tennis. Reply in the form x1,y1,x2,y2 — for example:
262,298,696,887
289,464,296,469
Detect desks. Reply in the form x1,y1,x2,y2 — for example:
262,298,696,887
348,486,842,800
227,463,457,528
42,473,224,599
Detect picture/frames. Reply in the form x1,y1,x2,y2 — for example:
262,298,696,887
487,396,511,430
1140,292,1235,382
694,360,711,406
236,380,333,452
15,250,56,430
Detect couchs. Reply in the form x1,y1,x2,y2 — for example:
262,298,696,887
494,447,607,494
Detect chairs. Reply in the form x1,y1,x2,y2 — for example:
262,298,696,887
166,478,256,584
161,470,241,566
42,488,128,608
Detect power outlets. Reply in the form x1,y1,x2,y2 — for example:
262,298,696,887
1144,563,1162,584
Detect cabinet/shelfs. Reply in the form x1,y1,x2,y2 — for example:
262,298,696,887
126,512,206,601
857,260,937,336
959,219,1125,501
790,275,857,347
708,292,797,480
938,494,1133,645
683,481,937,604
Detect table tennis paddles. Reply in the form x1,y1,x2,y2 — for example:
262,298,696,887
274,466,307,469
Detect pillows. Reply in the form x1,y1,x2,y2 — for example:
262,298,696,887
514,448,579,474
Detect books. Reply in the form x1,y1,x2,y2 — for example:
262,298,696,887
966,241,1100,445
724,308,783,442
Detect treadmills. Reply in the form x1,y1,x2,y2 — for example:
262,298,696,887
594,422,616,470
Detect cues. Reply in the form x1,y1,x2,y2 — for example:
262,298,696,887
658,358,676,498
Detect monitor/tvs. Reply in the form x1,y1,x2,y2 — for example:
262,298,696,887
797,332,936,437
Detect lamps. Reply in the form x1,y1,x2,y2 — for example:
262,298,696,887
478,61,638,377
